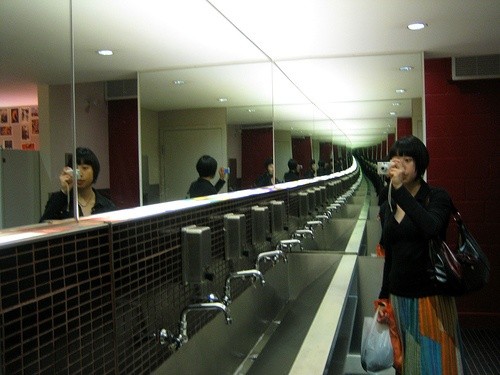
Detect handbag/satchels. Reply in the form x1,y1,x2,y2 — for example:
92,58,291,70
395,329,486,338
373,299,404,371
424,225,492,295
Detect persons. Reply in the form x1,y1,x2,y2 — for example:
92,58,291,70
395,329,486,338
184,154,227,200
253,158,286,188
38,147,121,224
304,156,353,178
372,133,470,374
283,158,301,183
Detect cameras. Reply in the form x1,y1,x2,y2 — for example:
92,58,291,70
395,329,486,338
65,167,80,180
377,160,396,175
224,168,230,174
299,165,302,169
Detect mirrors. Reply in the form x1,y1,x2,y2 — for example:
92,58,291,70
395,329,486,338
334,134,346,173
273,54,427,253
227,105,274,191
0,0,74,233
71,0,274,221
139,60,273,206
274,119,313,184
313,128,333,177
274,98,425,253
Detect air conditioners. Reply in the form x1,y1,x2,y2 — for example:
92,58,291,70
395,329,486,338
102,76,138,100
451,53,499,82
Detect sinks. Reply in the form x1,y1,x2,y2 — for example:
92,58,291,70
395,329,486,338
152,254,358,375
300,220,368,255
346,196,372,205
331,204,369,220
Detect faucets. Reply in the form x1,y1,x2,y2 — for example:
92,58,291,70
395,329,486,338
327,206,337,211
223,268,265,305
292,228,315,241
158,302,233,354
314,214,330,225
254,249,288,270
318,210,331,218
337,198,346,202
334,201,345,206
277,238,304,253
304,220,324,232
329,204,341,208
